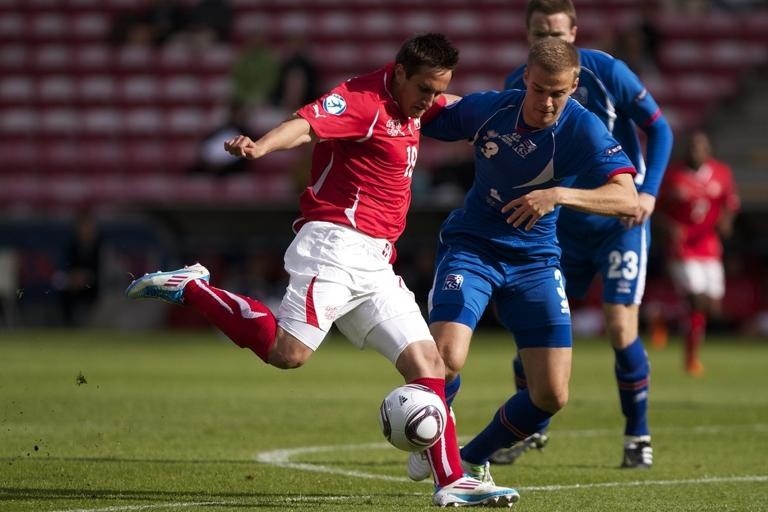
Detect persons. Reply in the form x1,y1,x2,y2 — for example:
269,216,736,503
485,0,674,467
3,218,768,342
399,32,638,503
647,125,742,376
121,34,525,509
242,57,321,163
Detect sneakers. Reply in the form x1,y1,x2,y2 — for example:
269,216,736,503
123,263,211,306
407,429,548,508
619,439,652,469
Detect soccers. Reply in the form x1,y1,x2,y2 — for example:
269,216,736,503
377,383,446,452
